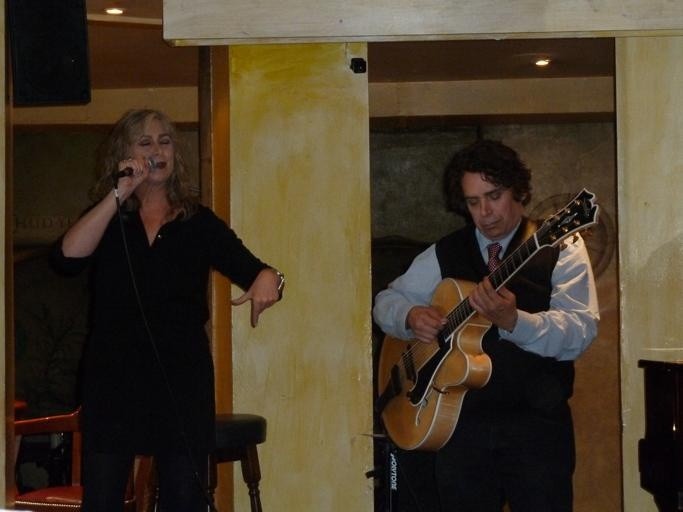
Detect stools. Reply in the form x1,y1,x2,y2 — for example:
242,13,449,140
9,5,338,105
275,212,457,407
147,415,266,511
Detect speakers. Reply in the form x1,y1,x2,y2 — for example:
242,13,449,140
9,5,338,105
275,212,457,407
4,0,92,107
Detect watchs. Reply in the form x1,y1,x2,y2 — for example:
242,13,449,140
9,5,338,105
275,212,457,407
277,271,286,298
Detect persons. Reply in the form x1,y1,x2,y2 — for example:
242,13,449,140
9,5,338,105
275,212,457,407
46,107,288,512
373,140,601,512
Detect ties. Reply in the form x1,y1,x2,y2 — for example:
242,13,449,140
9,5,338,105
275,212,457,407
485,243,502,275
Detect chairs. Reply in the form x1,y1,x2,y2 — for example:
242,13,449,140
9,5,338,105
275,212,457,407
7,400,151,510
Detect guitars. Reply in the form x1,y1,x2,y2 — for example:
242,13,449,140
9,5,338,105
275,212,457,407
378,187,601,452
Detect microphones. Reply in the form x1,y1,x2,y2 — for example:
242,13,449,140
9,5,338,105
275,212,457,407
111,156,156,178
